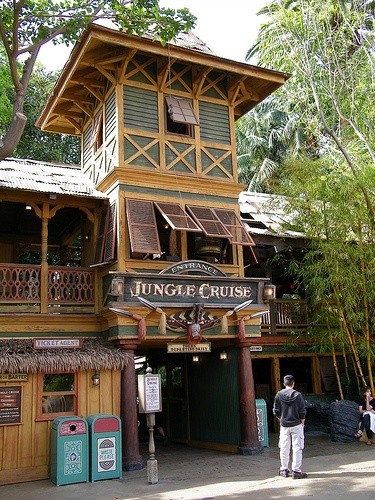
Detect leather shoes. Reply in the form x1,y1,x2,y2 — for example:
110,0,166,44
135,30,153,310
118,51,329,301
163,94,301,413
292,471,307,478
279,469,289,477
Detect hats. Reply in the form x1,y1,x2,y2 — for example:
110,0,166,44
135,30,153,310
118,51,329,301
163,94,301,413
284,375,294,380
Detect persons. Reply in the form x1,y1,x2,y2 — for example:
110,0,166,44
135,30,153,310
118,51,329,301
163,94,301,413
355,387,374,448
272,375,307,478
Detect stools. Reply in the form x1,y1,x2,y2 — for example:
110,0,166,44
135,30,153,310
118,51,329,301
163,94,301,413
359,422,375,444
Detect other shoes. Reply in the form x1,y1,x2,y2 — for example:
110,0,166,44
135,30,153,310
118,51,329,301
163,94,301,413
366,440,372,445
353,433,362,438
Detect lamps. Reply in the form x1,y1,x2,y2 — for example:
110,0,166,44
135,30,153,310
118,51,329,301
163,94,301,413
93,370,100,385
109,277,124,298
193,352,228,361
263,285,276,300
